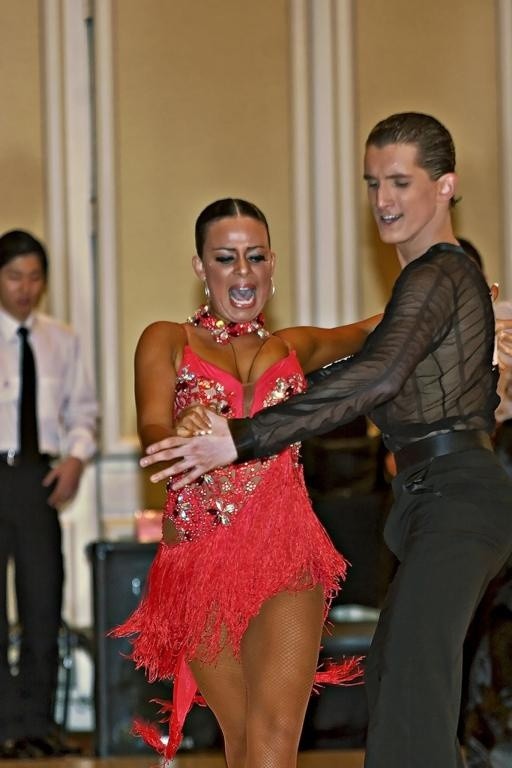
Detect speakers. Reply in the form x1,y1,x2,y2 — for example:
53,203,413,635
300,438,399,607
85,543,220,756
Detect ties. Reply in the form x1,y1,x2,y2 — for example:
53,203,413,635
17,327,37,470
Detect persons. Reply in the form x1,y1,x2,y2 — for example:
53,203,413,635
0,231,100,757
139,112,512,768
107,198,499,768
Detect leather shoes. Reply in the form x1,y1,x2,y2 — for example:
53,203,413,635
4,734,82,757
